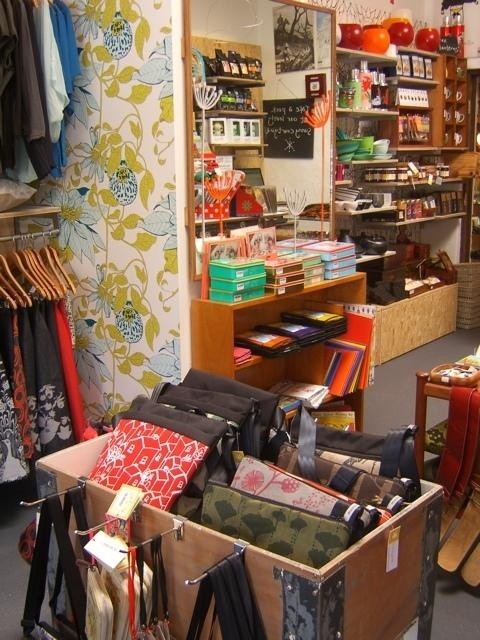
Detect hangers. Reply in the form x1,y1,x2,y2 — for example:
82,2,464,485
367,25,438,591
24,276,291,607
0,226,78,312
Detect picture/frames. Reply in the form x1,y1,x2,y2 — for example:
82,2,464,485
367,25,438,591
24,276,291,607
244,225,283,262
201,234,247,302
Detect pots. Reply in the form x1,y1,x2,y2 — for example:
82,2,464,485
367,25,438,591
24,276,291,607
362,235,387,254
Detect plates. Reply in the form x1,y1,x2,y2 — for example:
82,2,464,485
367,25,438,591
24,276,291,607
372,155,393,160
430,363,480,387
352,154,373,159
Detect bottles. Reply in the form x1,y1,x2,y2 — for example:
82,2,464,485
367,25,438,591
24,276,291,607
398,198,423,221
338,228,355,244
350,68,390,110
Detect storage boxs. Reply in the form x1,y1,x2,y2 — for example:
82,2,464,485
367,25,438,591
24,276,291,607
34,423,442,639
359,241,457,306
198,186,232,219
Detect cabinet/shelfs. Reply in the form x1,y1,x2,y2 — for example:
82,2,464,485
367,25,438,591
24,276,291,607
394,41,444,152
338,46,402,168
192,271,368,446
338,176,467,231
191,70,270,153
441,52,471,149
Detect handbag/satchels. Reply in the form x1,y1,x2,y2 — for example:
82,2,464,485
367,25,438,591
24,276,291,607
21,485,177,640
200,404,420,569
89,368,281,518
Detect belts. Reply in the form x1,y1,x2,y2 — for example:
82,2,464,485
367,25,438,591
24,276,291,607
435,387,480,587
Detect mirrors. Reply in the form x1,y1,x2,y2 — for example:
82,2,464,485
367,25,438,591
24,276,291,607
182,2,336,281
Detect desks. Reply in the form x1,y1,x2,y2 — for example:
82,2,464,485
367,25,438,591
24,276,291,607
410,355,480,480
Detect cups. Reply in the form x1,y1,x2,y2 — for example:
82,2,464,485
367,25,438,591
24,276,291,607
343,81,361,108
356,135,375,153
335,139,362,162
444,65,465,147
335,199,373,213
374,138,392,155
360,193,385,208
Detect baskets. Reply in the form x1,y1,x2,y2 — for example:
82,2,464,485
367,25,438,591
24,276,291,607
455,263,479,330
